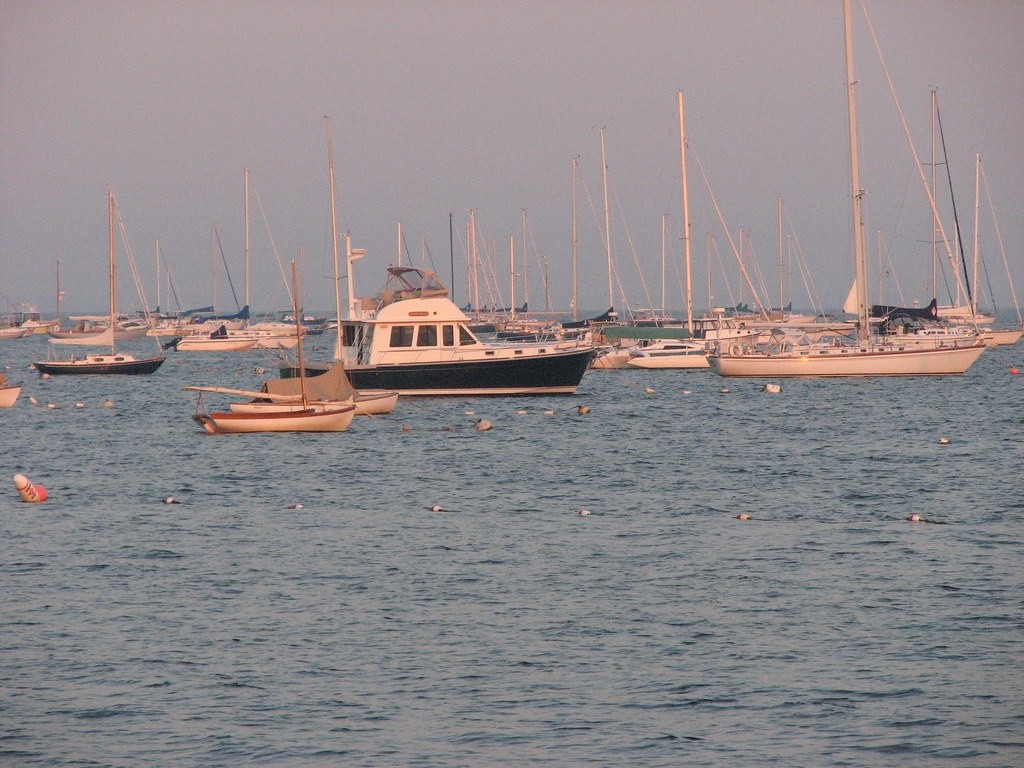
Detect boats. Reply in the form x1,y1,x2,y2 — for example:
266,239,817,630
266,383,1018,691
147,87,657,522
0,2,1023,378
0,372,22,410
275,212,596,398
179,256,361,434
228,112,398,417
